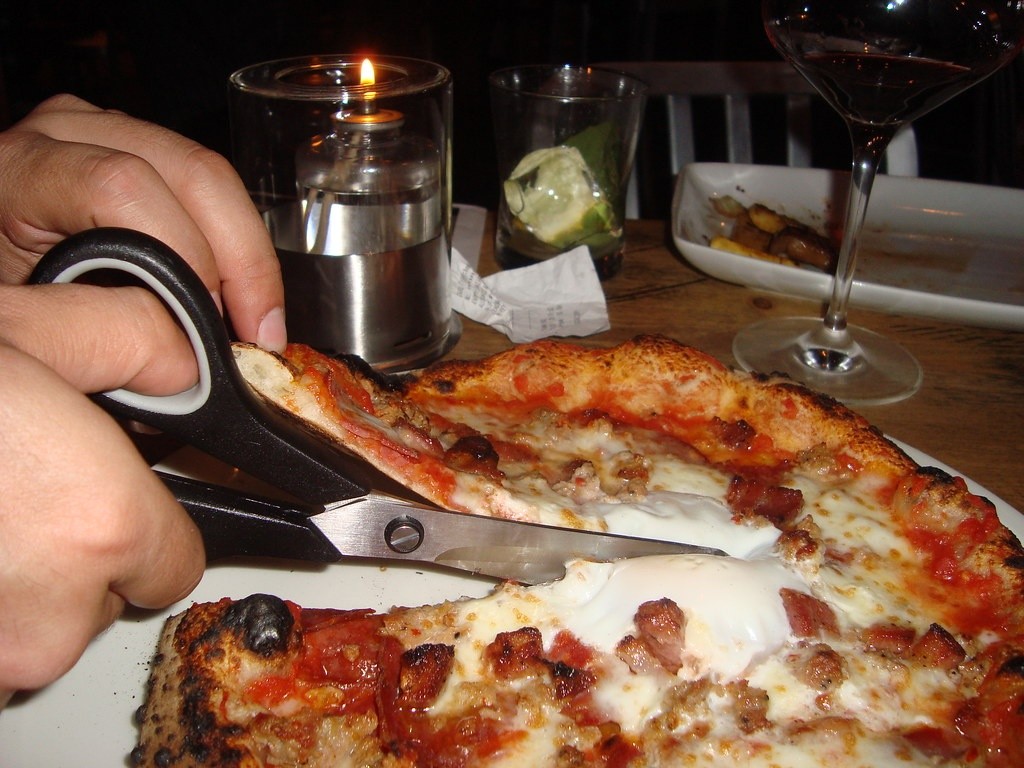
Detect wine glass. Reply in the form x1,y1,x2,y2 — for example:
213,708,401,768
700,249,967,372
730,0,1024,405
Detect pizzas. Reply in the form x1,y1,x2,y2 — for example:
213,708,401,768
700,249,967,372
129,328,1024,768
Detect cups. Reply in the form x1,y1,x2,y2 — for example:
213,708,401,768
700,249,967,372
489,66,651,279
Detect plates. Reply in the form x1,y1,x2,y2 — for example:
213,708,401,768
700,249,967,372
672,162,1024,328
0,435,1024,768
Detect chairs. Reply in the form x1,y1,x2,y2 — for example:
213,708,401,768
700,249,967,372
524,60,919,221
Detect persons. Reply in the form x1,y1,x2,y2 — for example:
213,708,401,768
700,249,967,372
0,92,285,706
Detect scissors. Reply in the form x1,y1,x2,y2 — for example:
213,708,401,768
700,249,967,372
18,224,733,587
